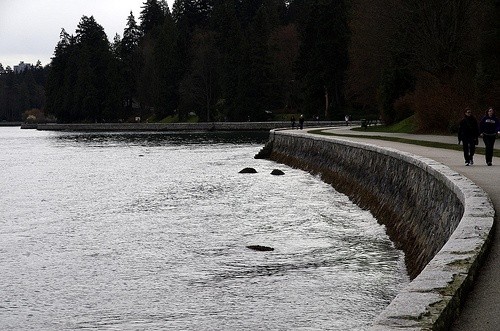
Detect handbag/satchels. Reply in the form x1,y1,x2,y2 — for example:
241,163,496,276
472,134,478,145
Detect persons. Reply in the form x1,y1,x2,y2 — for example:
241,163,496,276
299,115,304,129
481,107,500,166
291,115,295,129
345,115,349,126
458,107,480,166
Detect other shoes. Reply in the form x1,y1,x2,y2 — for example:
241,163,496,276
487,162,492,166
465,163,469,166
470,160,473,164
